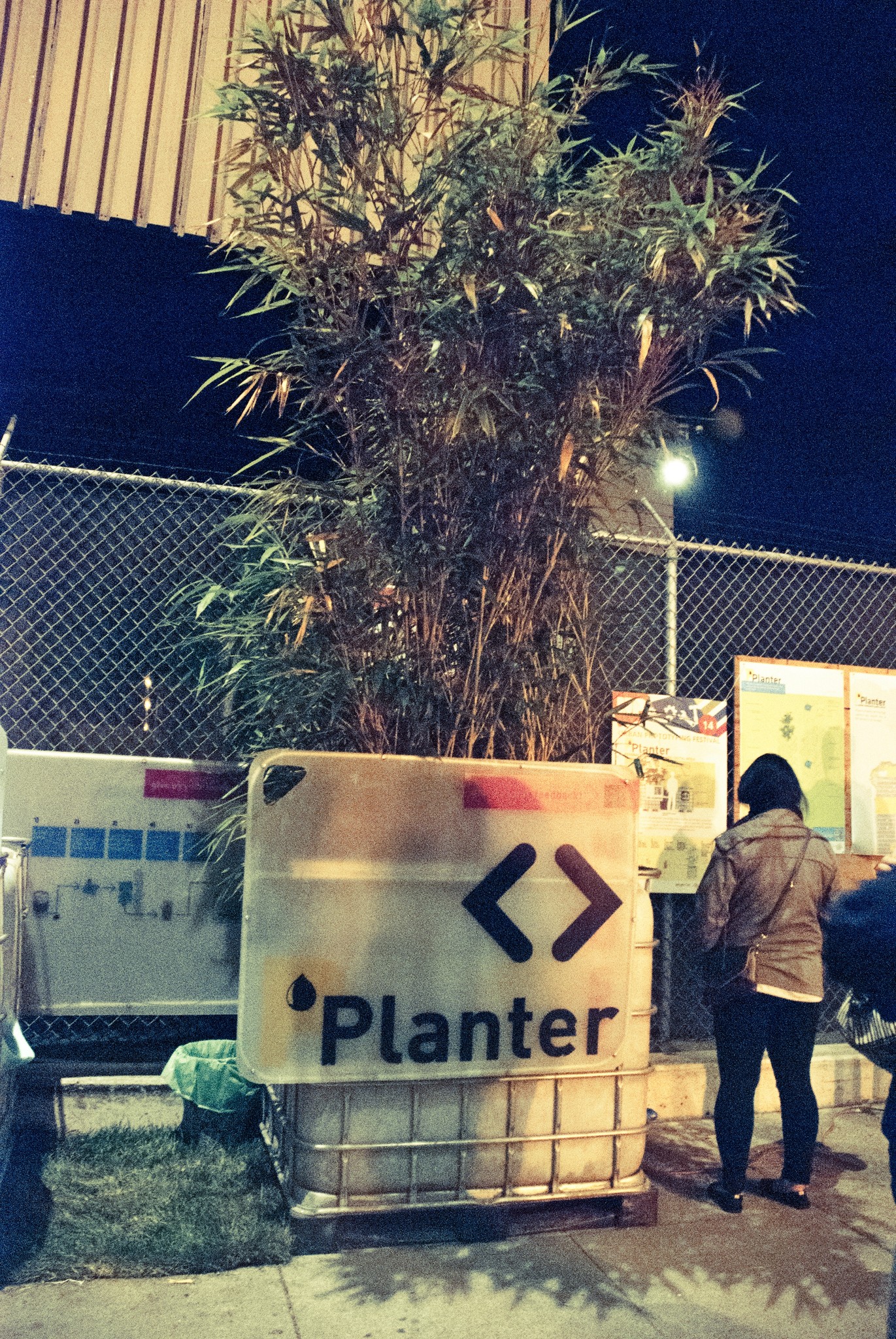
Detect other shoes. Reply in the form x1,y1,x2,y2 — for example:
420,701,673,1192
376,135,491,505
760,1176,809,1209
709,1181,743,1213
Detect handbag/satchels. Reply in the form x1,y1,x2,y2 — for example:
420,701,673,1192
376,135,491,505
703,945,757,999
830,987,896,1075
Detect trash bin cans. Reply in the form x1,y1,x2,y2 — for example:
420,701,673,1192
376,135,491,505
177,1040,263,1145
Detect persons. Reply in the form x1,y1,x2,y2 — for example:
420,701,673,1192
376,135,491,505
695,754,841,1215
821,853,896,1202
